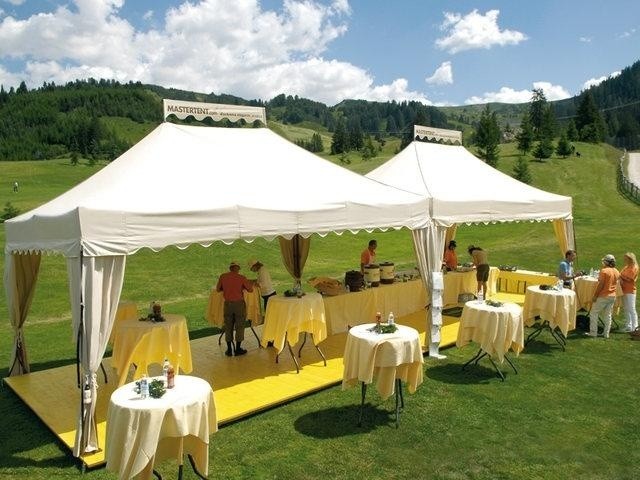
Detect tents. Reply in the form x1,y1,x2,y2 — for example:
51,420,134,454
3,99,433,457
362,125,574,353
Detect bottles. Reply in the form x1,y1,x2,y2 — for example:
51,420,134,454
376,312,381,324
345,284,350,293
153,302,161,315
590,267,594,277
167,365,175,389
557,279,563,291
163,359,169,380
140,374,147,400
388,312,394,324
150,301,155,313
296,281,302,298
477,289,483,303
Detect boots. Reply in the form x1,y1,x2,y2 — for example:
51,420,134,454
236,341,247,355
224,341,232,355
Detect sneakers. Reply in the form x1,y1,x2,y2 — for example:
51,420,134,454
585,333,597,337
599,332,610,338
620,327,634,332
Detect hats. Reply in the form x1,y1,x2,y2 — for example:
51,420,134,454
450,240,456,246
248,258,258,269
230,260,241,269
468,244,476,250
601,254,616,262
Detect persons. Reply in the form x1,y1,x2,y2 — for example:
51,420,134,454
584,254,620,338
361,241,376,274
248,258,277,311
216,262,253,357
464,244,490,301
443,240,457,270
620,252,640,331
559,250,581,288
